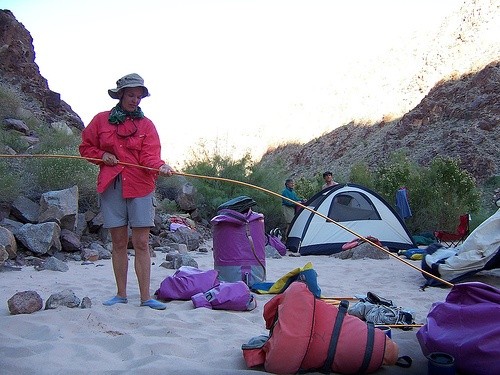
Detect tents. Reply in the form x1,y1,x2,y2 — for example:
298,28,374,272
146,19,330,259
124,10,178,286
285,183,419,256
419,208,500,287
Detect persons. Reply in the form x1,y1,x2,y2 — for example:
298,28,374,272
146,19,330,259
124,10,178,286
322,171,337,190
78,72,175,310
282,179,307,232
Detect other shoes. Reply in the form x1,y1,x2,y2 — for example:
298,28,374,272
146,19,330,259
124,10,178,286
139,297,167,310
102,296,128,306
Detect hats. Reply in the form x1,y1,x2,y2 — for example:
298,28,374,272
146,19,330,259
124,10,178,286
108,73,149,99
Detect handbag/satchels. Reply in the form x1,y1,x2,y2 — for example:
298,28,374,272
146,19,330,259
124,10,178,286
210,208,266,288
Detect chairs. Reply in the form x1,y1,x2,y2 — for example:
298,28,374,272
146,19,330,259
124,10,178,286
434,213,471,248
